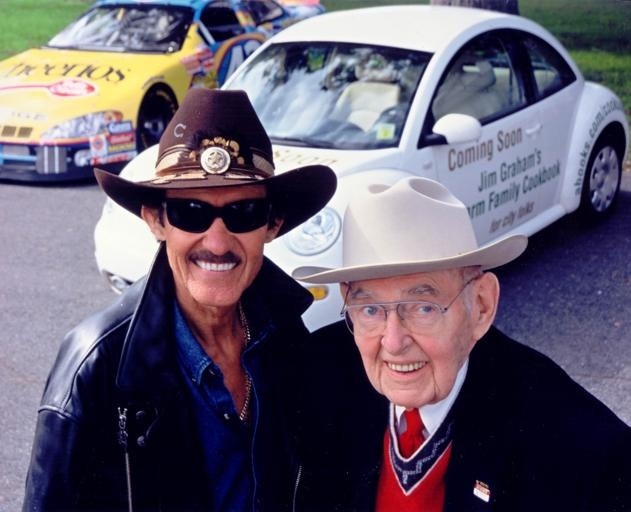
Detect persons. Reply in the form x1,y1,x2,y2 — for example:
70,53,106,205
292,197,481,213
19,87,339,512
291,169,629,511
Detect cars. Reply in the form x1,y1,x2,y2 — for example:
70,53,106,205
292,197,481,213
94,2,631,334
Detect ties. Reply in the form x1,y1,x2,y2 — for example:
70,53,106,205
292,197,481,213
399,408,425,459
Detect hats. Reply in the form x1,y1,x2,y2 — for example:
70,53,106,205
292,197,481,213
292,175,528,285
91,86,339,241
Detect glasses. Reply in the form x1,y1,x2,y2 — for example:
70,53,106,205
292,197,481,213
341,279,480,338
160,198,274,234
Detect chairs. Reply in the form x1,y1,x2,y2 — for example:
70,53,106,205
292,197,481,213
434,63,506,126
257,70,400,141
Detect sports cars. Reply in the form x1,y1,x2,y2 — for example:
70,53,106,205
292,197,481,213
1,1,326,183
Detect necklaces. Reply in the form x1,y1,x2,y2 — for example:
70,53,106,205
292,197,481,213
236,301,253,418
